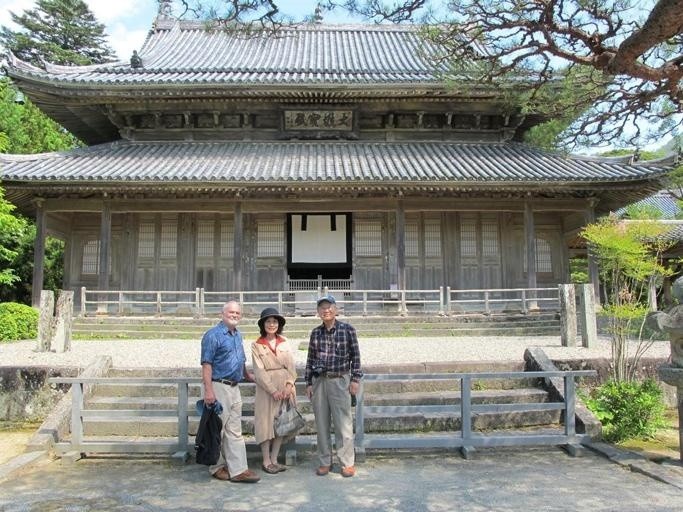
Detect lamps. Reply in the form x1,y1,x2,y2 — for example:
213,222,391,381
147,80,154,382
14,88,26,105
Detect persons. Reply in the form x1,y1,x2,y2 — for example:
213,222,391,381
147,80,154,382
200,300,262,484
304,295,363,477
251,307,298,474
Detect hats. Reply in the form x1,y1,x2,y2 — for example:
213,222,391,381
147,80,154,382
317,295,335,304
196,399,223,415
257,308,285,327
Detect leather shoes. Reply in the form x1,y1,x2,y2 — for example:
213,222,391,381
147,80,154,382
231,470,260,481
262,464,277,473
273,463,286,471
341,467,354,476
317,466,332,475
213,468,229,480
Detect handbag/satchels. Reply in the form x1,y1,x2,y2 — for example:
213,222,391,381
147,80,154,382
274,399,304,437
194,404,222,464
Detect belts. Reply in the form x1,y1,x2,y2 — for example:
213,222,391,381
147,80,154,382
212,378,236,385
320,371,348,378
